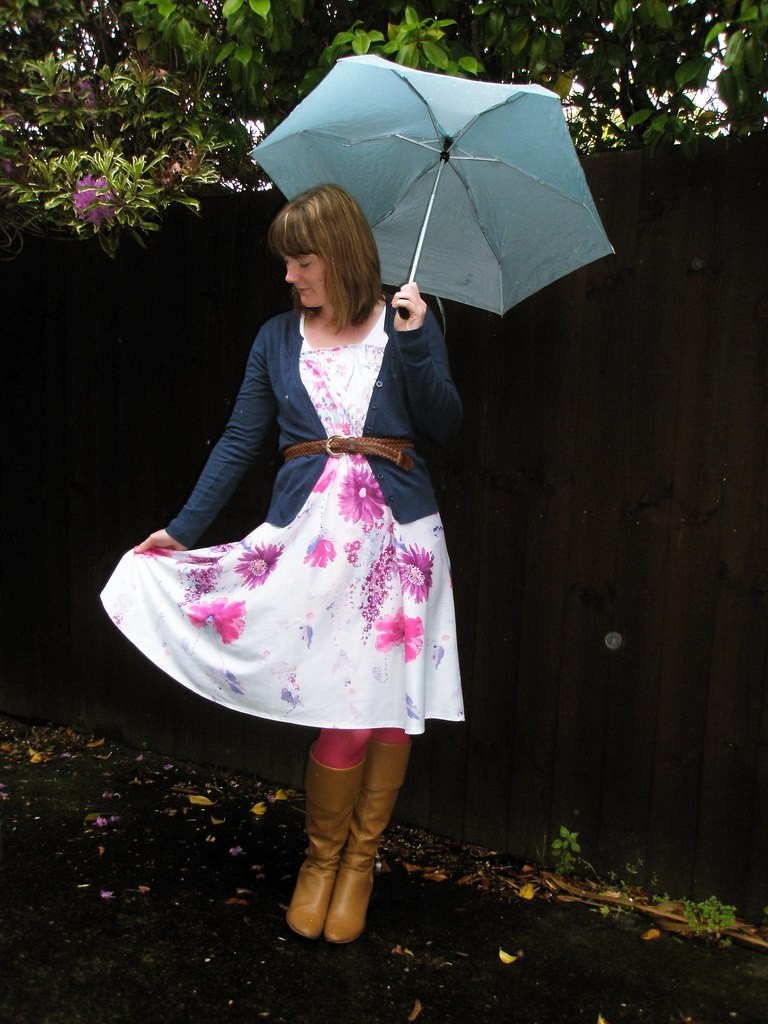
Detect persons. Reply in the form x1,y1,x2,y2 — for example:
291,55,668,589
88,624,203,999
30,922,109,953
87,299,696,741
99,182,466,946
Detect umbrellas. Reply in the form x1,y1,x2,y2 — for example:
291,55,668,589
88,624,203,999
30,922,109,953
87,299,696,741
247,52,618,320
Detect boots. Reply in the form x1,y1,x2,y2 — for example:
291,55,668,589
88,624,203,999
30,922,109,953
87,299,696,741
323,739,412,943
286,747,367,938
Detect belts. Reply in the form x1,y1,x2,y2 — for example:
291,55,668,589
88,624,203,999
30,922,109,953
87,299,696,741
284,435,415,471
